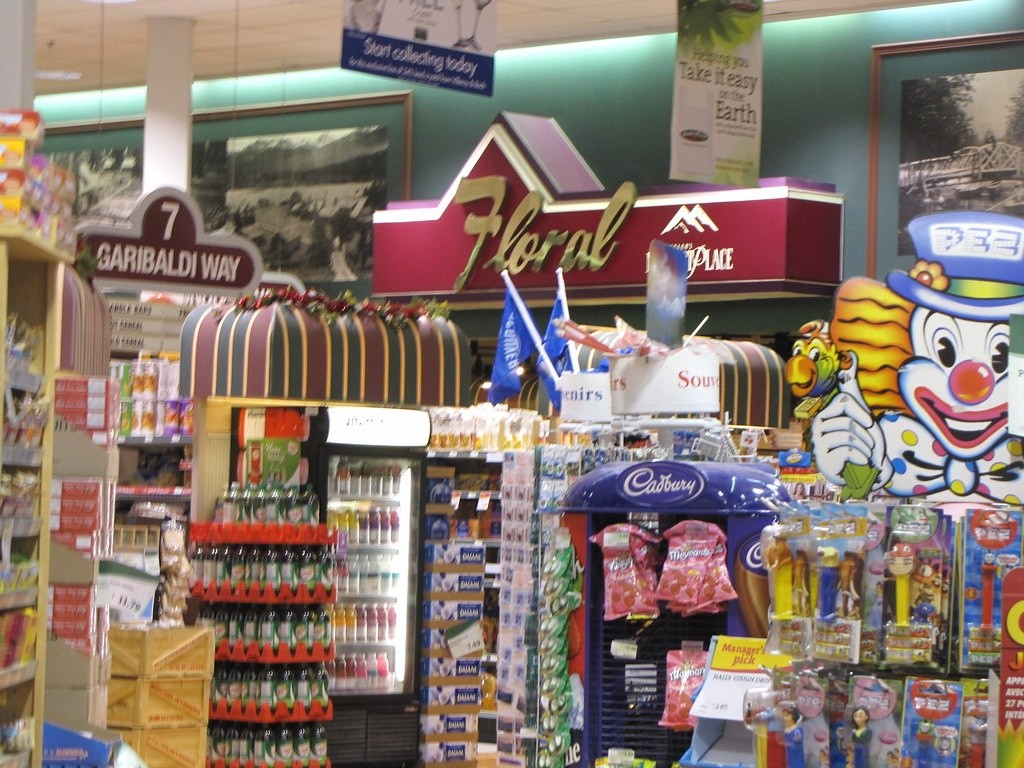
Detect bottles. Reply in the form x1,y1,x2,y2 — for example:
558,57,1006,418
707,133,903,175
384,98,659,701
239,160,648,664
326,652,390,689
213,480,321,527
326,503,399,544
211,661,329,710
186,542,335,593
334,601,397,642
332,454,404,498
207,721,327,767
337,551,396,594
194,601,331,650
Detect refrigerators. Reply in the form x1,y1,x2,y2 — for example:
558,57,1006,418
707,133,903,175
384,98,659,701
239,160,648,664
303,406,432,766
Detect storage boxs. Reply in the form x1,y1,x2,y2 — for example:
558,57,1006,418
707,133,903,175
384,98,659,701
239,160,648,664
419,464,488,768
40,372,163,768
106,625,215,768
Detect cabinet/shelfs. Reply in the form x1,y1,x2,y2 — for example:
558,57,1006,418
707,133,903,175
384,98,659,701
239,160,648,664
0,224,78,768
188,518,339,768
94,280,194,633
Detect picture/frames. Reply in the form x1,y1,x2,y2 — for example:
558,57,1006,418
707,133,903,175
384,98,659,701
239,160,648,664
864,28,1024,280
30,89,413,285
